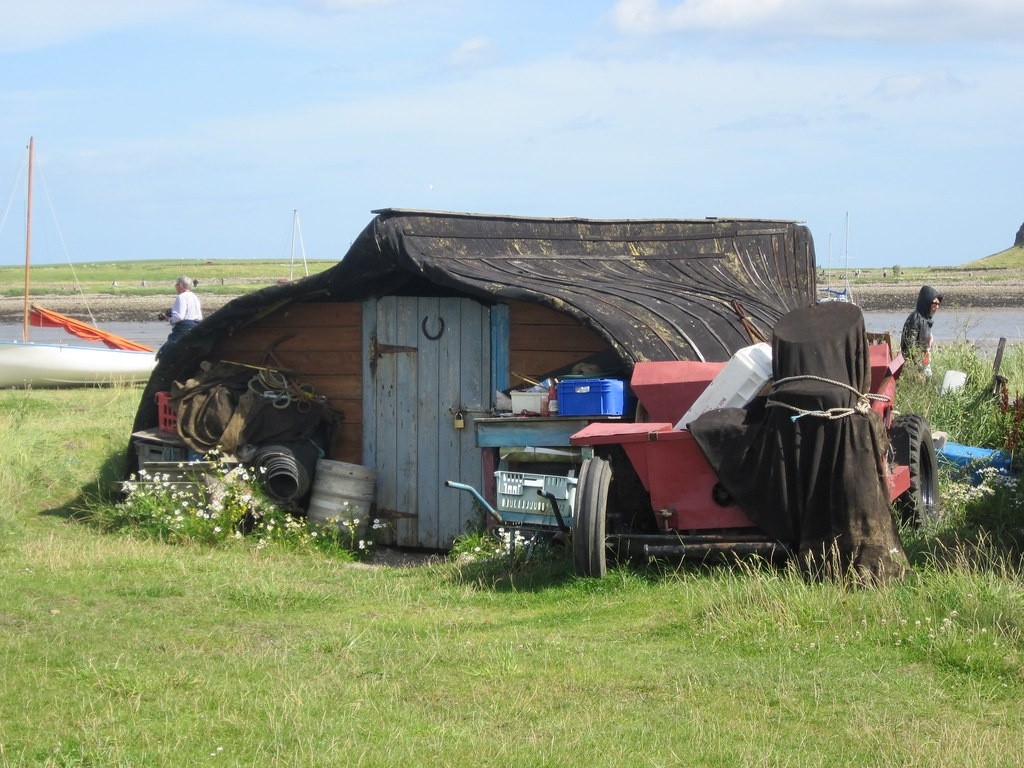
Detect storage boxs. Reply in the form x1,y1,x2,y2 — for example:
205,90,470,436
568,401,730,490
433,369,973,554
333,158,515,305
133,439,184,470
154,390,178,435
492,469,579,518
511,390,552,417
556,378,636,416
673,341,775,431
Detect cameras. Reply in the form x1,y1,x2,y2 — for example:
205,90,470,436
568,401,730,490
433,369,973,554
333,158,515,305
157,308,172,321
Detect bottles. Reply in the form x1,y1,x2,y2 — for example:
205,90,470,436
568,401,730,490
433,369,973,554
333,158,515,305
547,380,559,417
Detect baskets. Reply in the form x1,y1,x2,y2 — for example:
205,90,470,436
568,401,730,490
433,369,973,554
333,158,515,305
156,390,178,435
494,471,580,519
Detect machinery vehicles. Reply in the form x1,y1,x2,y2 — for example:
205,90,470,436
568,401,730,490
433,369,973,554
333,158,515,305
565,334,942,579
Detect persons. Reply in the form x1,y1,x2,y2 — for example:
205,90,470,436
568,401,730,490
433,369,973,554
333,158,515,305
901,286,943,390
158,277,203,363
854,265,888,279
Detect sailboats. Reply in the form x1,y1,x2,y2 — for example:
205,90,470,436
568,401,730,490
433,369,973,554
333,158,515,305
815,212,855,304
0,139,160,391
